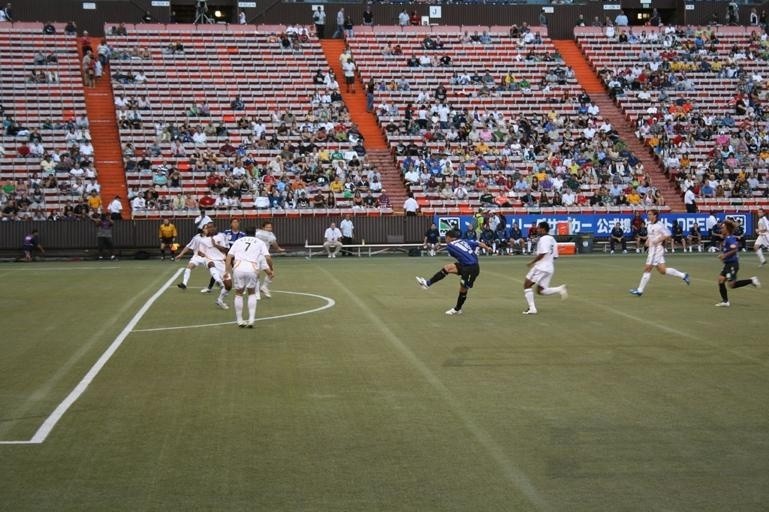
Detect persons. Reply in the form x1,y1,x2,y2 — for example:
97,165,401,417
200,217,247,293
416,231,494,315
628,210,690,296
521,221,569,315
1,0,769,263
715,220,761,307
176,224,209,288
197,222,232,310
225,227,275,329
255,222,285,299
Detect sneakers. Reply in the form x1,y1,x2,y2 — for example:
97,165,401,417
684,274,690,286
446,307,462,316
522,307,537,315
260,286,271,298
715,301,730,307
201,288,230,310
749,277,759,291
560,284,567,301
415,276,430,290
629,289,642,296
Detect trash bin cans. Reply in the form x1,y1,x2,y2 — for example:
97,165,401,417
578,235,593,253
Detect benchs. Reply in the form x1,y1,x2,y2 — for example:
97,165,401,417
302,234,769,258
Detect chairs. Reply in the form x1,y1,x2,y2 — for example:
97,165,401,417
0,13,769,218
